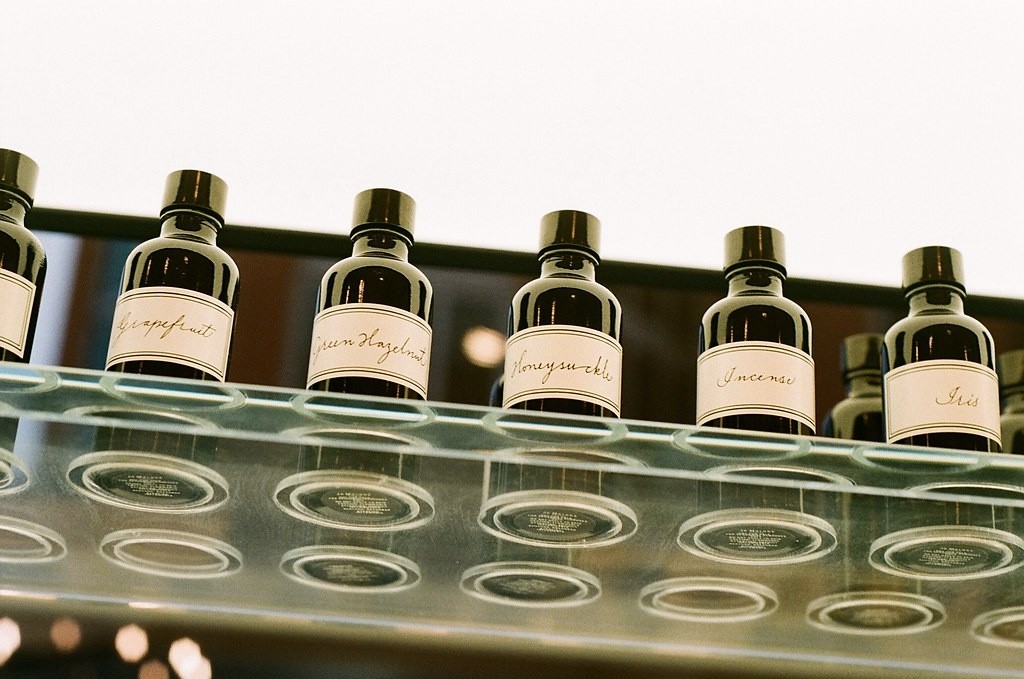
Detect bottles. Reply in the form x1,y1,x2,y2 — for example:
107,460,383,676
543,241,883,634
88,169,238,510
821,335,925,633
880,245,1004,578
986,352,1024,641
696,226,815,559
1,148,47,485
296,188,623,603
